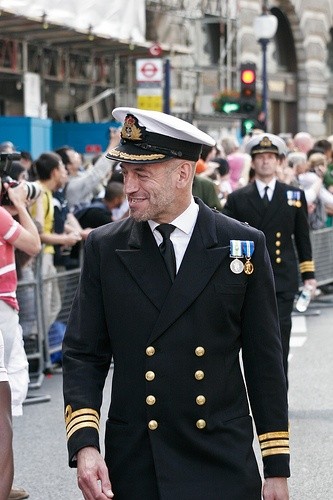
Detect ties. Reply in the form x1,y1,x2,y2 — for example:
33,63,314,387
156,224,177,285
261,186,271,213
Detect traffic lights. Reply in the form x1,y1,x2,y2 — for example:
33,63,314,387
217,60,258,138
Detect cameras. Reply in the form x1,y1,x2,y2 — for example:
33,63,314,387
0,142,42,206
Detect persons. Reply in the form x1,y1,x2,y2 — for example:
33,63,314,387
0,131,333,400
62,106,292,500
0,159,42,500
219,133,319,390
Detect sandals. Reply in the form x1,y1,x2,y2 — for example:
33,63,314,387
7,488,29,500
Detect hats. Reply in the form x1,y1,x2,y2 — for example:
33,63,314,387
107,107,217,167
247,133,289,158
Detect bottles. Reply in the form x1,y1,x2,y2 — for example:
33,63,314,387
296,286,313,312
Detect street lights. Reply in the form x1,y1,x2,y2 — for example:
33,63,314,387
253,7,279,134
